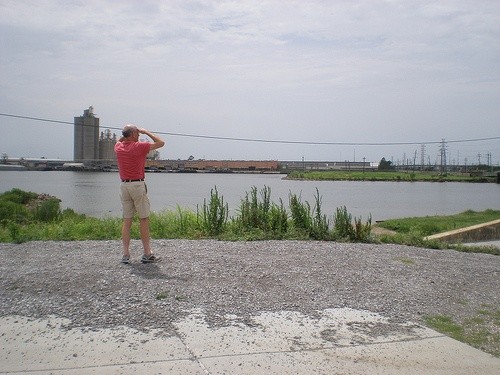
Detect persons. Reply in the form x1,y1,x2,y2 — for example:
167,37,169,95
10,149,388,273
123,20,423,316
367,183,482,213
114,125,165,263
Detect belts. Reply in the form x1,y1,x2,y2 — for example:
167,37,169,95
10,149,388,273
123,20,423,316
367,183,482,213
122,179,144,182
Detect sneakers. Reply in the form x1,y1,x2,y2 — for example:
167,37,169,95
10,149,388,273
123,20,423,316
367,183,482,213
122,255,131,263
141,254,163,263
